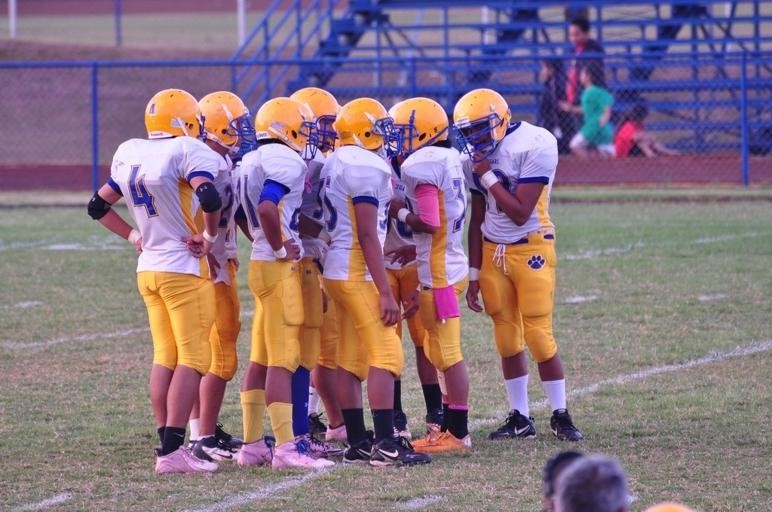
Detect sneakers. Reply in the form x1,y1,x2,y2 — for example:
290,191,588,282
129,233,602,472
488,409,536,440
550,409,584,441
408,425,444,448
412,429,472,454
155,411,432,477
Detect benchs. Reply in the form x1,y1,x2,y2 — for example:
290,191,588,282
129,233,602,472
267,1,771,159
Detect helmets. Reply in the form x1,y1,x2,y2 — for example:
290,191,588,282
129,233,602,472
337,97,390,150
144,88,205,144
255,97,320,160
454,88,512,154
291,86,342,152
388,97,450,162
197,90,256,149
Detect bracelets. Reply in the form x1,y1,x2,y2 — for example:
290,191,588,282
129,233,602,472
467,266,480,283
396,207,411,223
127,226,143,247
479,169,500,191
202,229,220,245
271,245,288,260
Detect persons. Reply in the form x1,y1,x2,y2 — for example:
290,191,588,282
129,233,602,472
86,86,229,472
453,88,584,441
309,371,350,446
388,96,478,455
567,60,620,158
532,56,567,153
387,150,449,441
533,446,634,511
316,94,434,469
290,85,347,462
563,16,606,133
188,89,258,467
611,102,681,161
234,95,337,470
